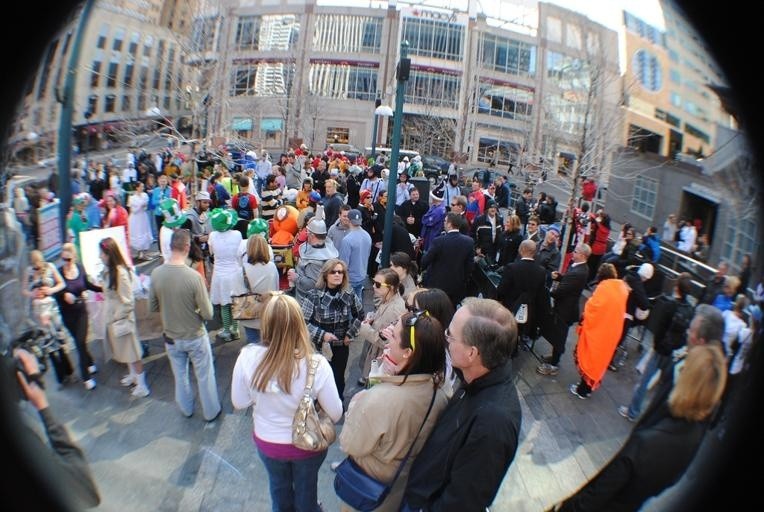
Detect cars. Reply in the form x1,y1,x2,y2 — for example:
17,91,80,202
220,141,274,162
7,173,35,187
324,138,513,191
36,152,57,166
599,225,728,310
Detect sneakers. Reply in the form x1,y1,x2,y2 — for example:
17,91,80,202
569,381,592,400
85,380,96,390
133,388,150,398
88,365,97,375
536,364,560,375
619,406,635,422
121,376,137,387
216,327,240,342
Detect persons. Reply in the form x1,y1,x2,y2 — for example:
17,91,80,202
2,144,764,512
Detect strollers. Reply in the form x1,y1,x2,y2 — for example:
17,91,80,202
470,253,547,365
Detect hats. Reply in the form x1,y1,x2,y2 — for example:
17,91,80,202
485,199,497,209
209,208,239,231
309,191,321,203
359,190,372,203
348,209,362,225
306,219,327,234
247,219,270,241
160,199,187,227
547,224,562,235
301,177,314,192
638,263,654,278
749,304,763,321
195,191,211,201
430,173,448,201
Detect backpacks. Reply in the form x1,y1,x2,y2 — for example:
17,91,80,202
508,263,543,336
655,302,695,356
238,193,251,219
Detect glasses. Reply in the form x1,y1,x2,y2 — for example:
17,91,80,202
407,310,429,352
444,329,463,345
372,279,389,288
328,271,344,274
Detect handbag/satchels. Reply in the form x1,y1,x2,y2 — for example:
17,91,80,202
291,398,336,452
335,456,390,512
113,320,133,337
231,293,263,320
